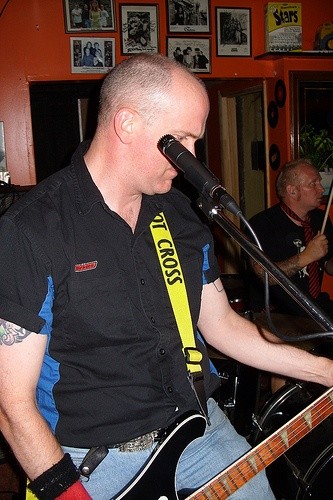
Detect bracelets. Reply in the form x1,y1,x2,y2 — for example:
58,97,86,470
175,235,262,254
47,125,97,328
27,453,94,500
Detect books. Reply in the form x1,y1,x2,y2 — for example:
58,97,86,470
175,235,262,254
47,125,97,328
264,2,302,53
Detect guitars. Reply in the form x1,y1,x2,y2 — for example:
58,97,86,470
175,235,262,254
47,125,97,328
112,383,333,499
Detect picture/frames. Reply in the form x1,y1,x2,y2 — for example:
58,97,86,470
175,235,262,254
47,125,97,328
214,5,251,58
118,2,160,57
70,35,117,74
165,0,213,74
64,0,117,34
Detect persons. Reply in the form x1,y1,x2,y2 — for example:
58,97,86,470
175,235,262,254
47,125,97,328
69,0,243,69
244,159,333,392
0,53,333,500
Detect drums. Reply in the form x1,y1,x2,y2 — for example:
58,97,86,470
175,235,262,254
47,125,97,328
250,377,333,499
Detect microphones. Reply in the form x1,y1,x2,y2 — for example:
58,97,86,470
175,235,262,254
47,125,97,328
157,134,242,216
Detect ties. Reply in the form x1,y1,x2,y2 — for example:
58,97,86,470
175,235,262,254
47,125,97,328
280,202,325,299
